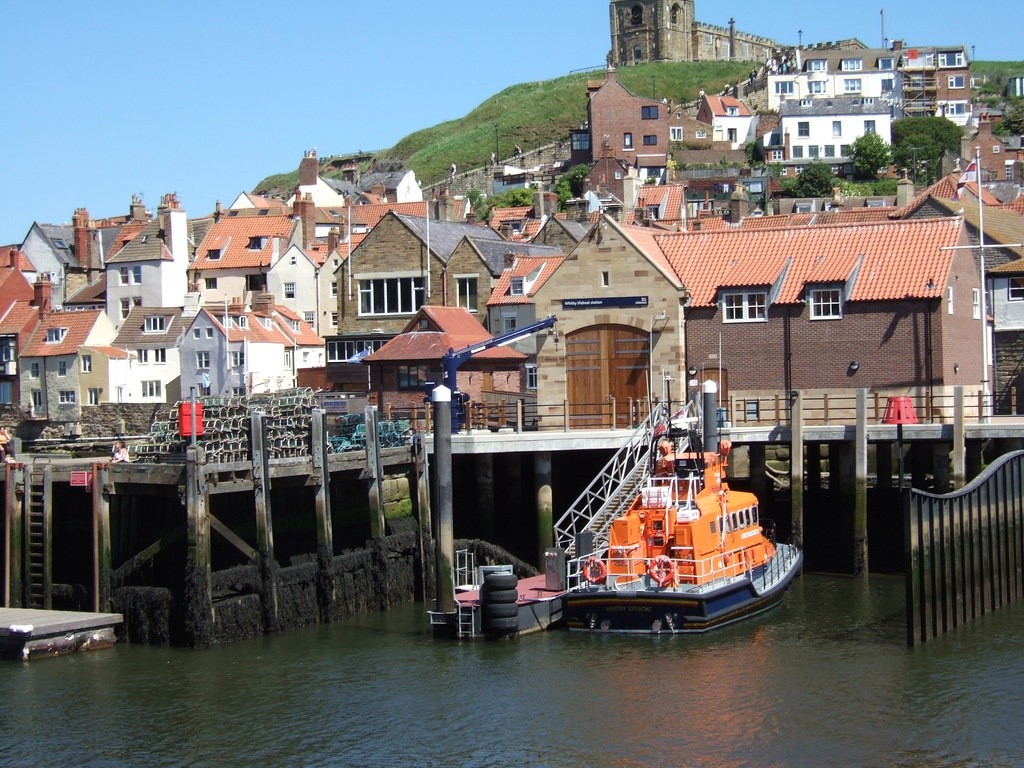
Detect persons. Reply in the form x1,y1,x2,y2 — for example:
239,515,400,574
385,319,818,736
0,425,16,464
747,66,758,86
490,151,497,169
448,162,457,180
513,142,522,159
698,87,705,100
105,439,130,464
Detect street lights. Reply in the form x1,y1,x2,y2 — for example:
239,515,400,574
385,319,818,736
495,124,499,164
912,148,923,187
971,45,975,58
798,30,803,49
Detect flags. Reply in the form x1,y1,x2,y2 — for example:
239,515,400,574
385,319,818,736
950,157,978,204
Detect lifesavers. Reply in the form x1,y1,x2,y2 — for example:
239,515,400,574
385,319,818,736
648,554,676,586
583,557,608,584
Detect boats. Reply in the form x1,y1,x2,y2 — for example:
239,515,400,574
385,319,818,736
563,371,805,634
